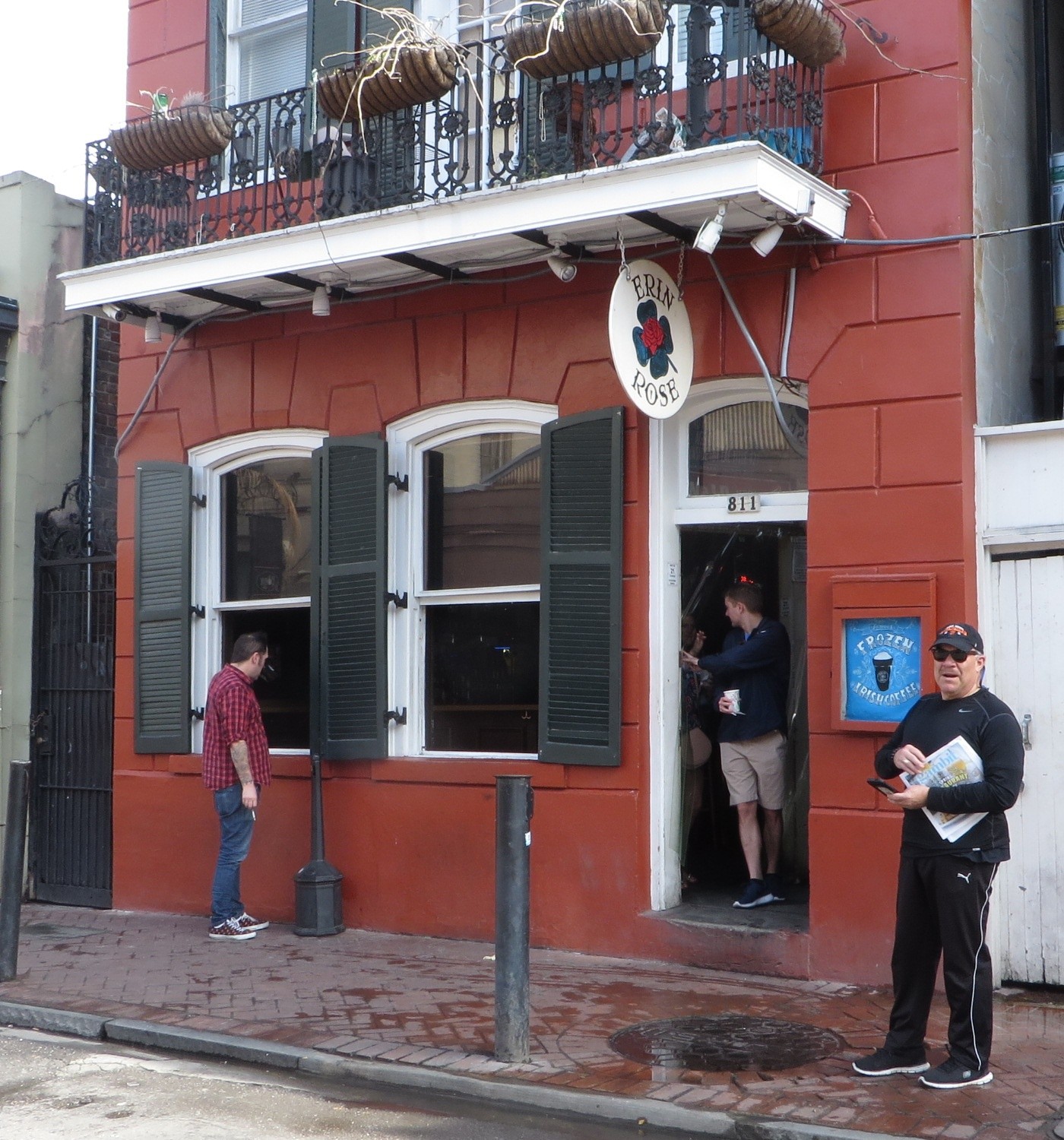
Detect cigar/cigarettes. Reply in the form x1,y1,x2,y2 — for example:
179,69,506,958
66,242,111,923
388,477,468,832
252,809,256,822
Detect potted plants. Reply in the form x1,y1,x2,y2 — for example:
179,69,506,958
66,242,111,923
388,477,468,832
304,0,509,170
483,1,667,77
108,83,236,173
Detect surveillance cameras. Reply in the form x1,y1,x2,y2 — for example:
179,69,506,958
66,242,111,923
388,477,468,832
102,304,130,321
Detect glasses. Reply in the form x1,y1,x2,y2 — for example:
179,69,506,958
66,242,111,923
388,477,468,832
931,648,978,663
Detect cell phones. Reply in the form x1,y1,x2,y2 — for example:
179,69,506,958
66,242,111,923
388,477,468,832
867,778,898,798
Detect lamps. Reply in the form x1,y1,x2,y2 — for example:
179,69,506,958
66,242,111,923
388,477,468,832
101,302,127,322
312,270,335,319
749,215,806,258
545,232,578,283
144,301,167,344
691,200,729,255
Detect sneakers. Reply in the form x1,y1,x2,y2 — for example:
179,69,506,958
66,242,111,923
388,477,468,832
732,883,773,908
209,919,256,940
236,911,270,931
767,886,786,900
917,1056,993,1089
852,1047,930,1076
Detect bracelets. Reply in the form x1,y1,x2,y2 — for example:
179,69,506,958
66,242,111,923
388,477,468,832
891,747,901,762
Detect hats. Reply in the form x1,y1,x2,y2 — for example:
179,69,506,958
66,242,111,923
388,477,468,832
928,622,984,653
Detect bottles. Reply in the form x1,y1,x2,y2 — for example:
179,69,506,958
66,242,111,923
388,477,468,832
150,92,167,121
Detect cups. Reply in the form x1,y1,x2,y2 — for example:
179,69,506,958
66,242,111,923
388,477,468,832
723,690,740,712
872,657,893,691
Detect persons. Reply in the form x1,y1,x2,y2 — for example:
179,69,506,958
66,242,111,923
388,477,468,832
201,634,270,941
851,622,1025,1090
682,581,788,907
681,615,712,891
